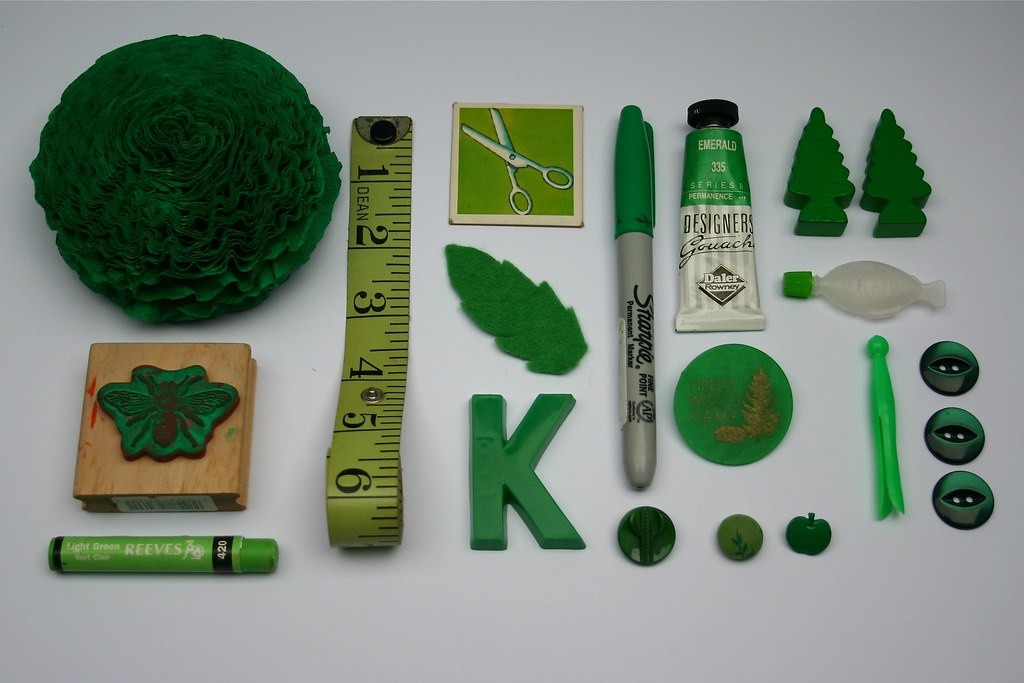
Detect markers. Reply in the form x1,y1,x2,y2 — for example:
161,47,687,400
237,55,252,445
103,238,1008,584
607,101,663,491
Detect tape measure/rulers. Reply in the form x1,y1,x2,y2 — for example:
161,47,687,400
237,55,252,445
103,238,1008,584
323,109,418,554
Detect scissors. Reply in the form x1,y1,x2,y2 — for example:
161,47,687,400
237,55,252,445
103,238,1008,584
461,107,574,214
